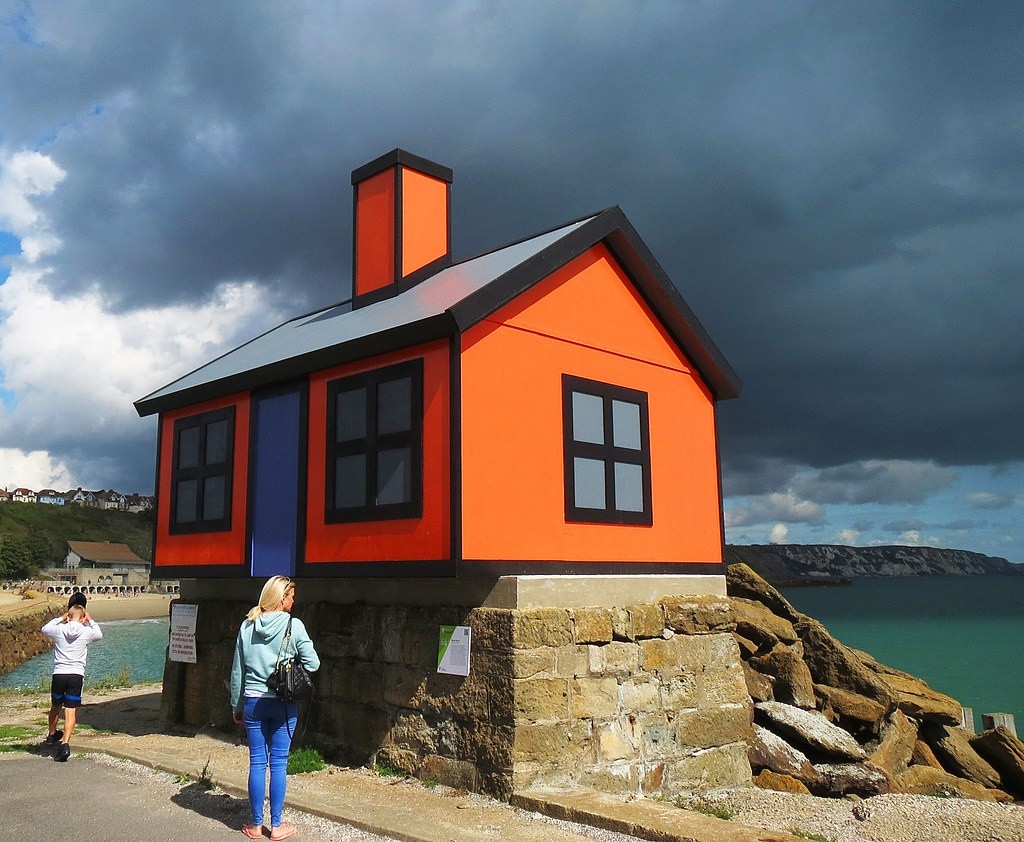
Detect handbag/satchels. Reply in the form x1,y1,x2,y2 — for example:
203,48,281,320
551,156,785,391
264,656,315,703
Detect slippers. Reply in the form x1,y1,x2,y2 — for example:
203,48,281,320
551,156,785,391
241,822,296,840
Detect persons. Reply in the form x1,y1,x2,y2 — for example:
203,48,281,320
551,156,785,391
230,575,320,841
42,603,104,763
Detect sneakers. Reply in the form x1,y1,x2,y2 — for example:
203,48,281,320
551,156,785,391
46,731,71,762
